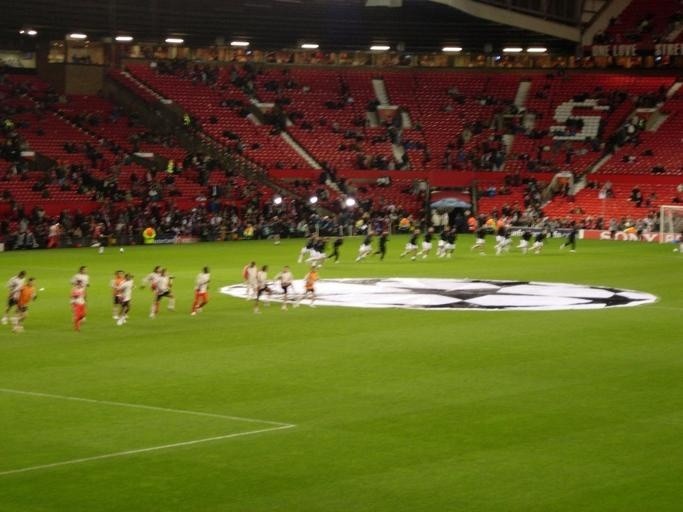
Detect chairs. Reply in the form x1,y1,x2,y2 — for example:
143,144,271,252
1,65,683,236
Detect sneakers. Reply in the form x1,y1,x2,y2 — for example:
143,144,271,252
1,305,205,334
295,244,578,268
241,292,318,316
